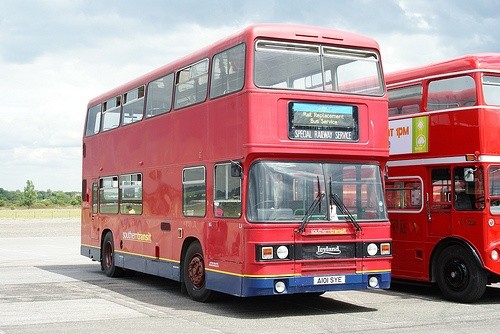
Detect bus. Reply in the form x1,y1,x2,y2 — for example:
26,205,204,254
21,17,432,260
339,53,500,303
79,23,392,303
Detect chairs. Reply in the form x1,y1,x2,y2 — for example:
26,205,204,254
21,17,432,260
455,192,472,209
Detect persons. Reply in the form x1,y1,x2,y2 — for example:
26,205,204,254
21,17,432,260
127,205,135,214
215,206,224,216
411,187,421,206
